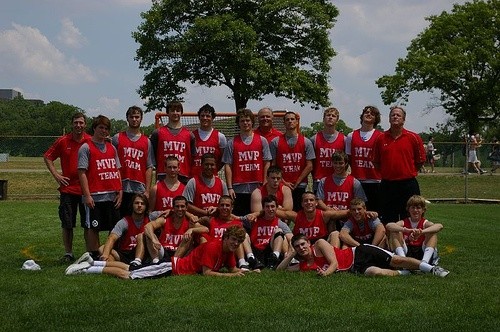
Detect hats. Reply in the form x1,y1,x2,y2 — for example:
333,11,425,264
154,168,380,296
20,260,41,271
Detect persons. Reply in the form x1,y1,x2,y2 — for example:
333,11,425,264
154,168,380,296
65,226,262,279
275,235,450,278
42,103,500,264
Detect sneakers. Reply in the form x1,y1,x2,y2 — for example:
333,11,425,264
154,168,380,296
58,254,73,265
129,259,142,271
66,261,90,275
150,259,163,265
433,258,441,265
430,267,450,278
88,251,100,260
410,270,422,275
74,251,93,264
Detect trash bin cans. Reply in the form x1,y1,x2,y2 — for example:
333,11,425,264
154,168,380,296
0,179,8,200
